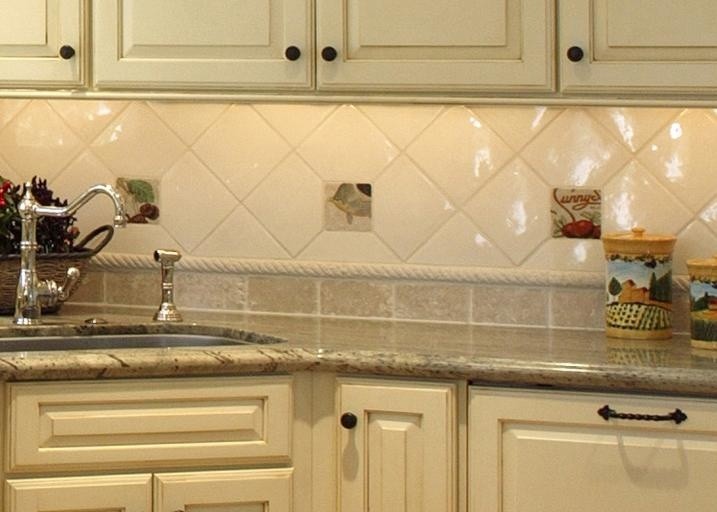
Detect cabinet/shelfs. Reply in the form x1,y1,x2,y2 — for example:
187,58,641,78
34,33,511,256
84,3,557,109
311,365,460,512
1,370,311,512
0,0,89,100
458,376,714,512
558,0,717,109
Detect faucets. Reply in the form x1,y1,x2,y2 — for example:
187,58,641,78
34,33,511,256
11,182,125,327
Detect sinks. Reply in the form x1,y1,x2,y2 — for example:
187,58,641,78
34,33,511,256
0,323,289,355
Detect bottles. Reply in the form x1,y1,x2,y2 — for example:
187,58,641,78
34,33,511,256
685,257,716,350
600,226,676,341
